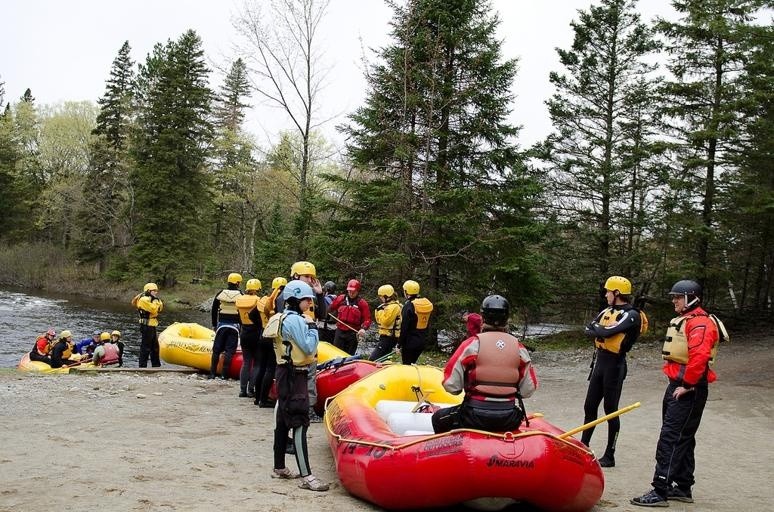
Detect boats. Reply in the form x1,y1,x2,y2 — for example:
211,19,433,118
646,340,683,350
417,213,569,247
158,321,254,379
323,363,603,509
266,340,382,416
19,350,101,372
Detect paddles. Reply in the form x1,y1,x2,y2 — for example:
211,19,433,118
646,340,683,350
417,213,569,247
46,358,93,374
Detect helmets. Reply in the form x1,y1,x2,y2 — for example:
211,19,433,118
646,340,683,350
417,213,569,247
60,331,71,340
228,273,243,285
100,332,111,341
91,330,101,336
290,261,316,278
604,276,632,295
144,283,158,293
346,279,361,292
378,284,394,298
244,279,262,292
480,295,510,327
272,277,287,290
282,280,315,300
46,329,56,336
668,280,702,296
403,280,420,295
324,281,336,292
111,330,121,337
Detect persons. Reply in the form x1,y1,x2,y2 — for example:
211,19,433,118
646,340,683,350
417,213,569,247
371,285,403,363
629,278,721,508
430,292,539,434
395,280,434,366
134,283,164,368
579,275,641,467
261,278,330,495
30,329,125,369
462,313,484,337
209,273,370,380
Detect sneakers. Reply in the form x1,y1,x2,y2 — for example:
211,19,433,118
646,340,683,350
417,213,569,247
271,467,301,479
298,475,329,491
630,490,669,507
668,487,692,502
598,459,615,467
